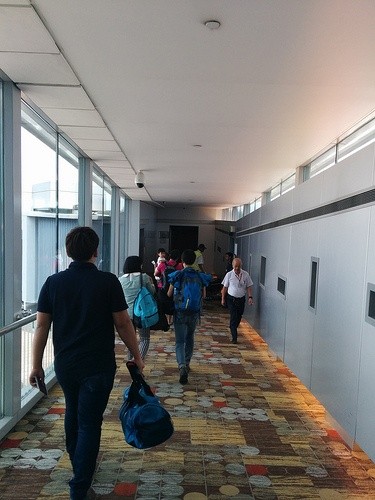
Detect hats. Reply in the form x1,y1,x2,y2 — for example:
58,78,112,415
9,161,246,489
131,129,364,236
198,244,207,250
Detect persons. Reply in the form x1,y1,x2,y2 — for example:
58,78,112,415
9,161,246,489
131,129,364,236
118,256,156,362
152,244,206,324
30,226,146,500
221,258,253,344
224,252,233,274
167,249,211,384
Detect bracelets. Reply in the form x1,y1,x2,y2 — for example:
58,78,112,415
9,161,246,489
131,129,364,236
249,296,252,298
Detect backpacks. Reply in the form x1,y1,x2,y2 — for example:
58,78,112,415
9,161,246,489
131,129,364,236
162,260,180,289
132,273,159,329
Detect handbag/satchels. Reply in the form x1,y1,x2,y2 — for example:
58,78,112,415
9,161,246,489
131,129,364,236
117,361,174,450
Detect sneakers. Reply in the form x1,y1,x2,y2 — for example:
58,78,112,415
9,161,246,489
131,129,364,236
84,487,96,500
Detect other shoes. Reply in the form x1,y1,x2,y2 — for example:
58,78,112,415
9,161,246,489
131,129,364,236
180,374,188,385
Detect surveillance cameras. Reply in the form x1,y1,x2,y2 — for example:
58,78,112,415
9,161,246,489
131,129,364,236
135,172,144,188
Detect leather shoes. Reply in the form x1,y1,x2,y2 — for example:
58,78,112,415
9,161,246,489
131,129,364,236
232,338,237,343
